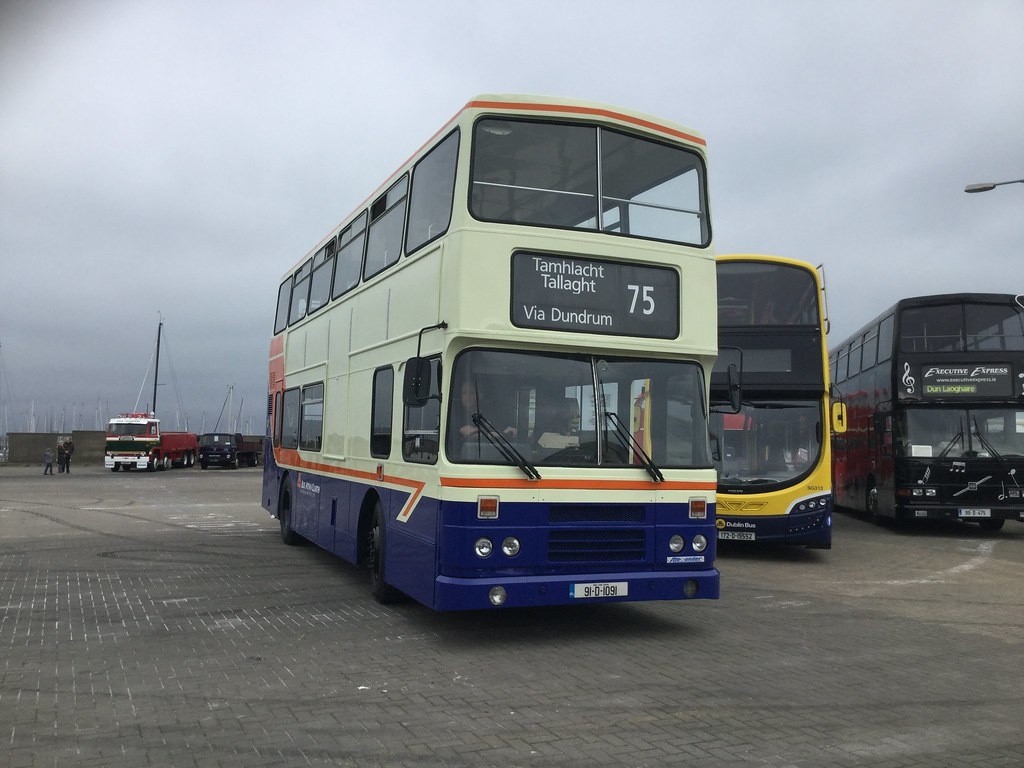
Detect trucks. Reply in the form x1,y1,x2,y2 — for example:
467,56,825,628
198,432,263,470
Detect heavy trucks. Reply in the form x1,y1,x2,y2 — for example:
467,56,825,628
101,409,198,473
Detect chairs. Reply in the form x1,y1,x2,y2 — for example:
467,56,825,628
428,221,445,240
385,248,390,266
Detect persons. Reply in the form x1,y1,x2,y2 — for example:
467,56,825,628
57,437,74,473
449,379,517,460
43,448,54,475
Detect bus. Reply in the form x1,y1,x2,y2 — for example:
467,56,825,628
259,95,741,619
631,251,849,552
828,290,1023,535
